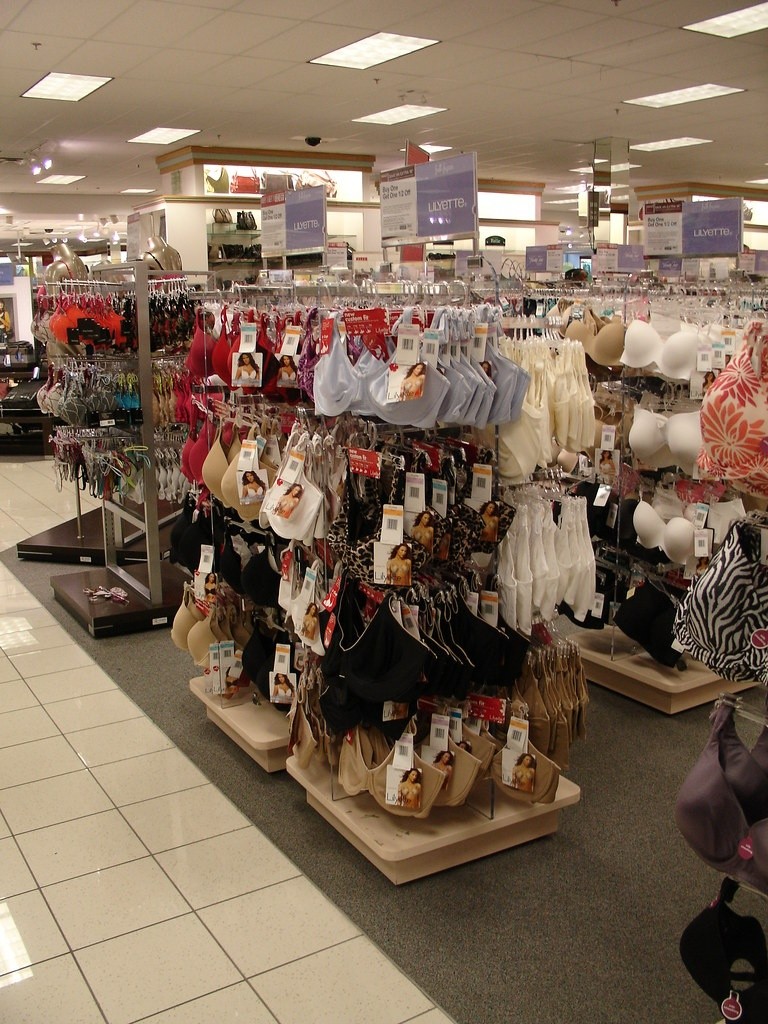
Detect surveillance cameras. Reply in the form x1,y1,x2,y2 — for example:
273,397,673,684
305,137,321,146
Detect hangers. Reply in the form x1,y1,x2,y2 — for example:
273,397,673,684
35,281,768,736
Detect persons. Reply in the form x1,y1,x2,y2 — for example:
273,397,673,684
397,768,422,808
44,242,92,297
225,668,240,693
509,754,538,791
143,235,181,293
478,502,497,541
202,575,216,603
482,361,493,378
411,511,435,547
233,353,259,386
241,471,266,499
278,355,298,387
395,703,407,718
0,299,11,342
386,544,411,585
399,362,427,400
303,600,318,639
275,484,302,519
272,673,295,703
433,741,474,791
703,356,730,398
573,269,590,285
600,450,616,484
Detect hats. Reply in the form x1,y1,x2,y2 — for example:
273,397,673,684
231,167,259,193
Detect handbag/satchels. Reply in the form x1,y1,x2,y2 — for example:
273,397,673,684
295,169,337,198
237,211,257,230
261,169,294,192
208,243,262,292
205,167,229,193
213,208,233,223
